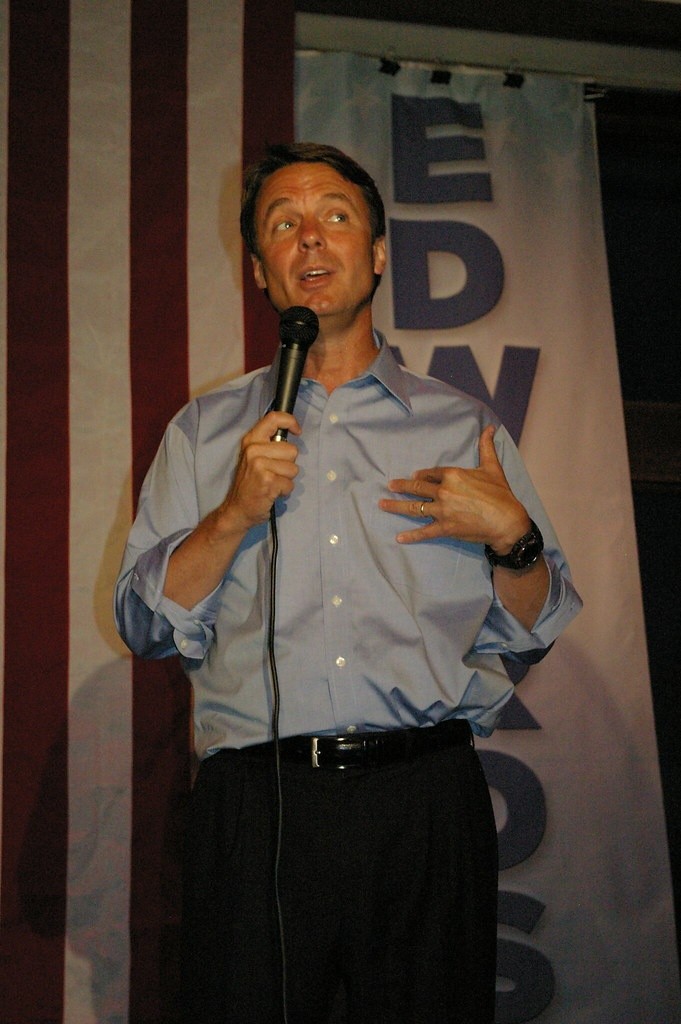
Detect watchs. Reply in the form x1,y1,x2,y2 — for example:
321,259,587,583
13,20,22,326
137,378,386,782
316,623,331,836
484,519,545,570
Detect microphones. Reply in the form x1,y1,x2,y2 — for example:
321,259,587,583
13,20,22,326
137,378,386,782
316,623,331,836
270,303,319,445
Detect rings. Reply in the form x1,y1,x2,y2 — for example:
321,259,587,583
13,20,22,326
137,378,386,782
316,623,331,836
420,503,428,516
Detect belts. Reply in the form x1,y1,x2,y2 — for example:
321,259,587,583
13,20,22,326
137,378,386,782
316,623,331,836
216,720,472,770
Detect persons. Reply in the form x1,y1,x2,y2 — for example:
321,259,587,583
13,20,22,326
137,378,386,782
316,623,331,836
111,142,585,1024
